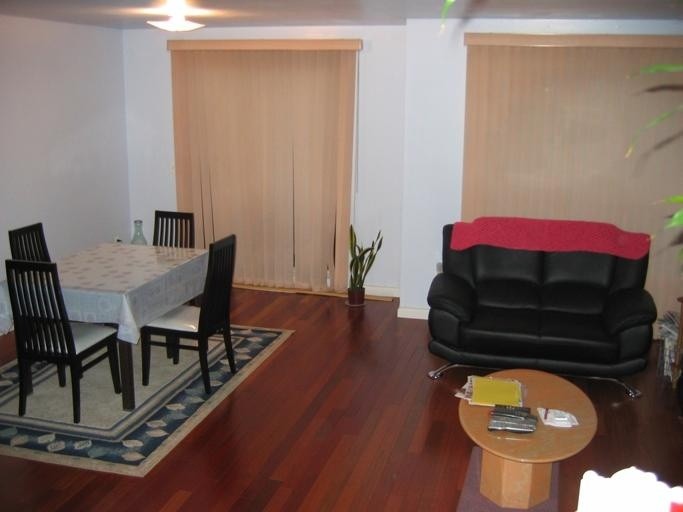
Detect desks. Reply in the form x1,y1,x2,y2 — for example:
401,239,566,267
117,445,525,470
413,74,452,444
13,242,208,411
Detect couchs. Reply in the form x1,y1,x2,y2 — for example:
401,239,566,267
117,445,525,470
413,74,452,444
421,217,654,404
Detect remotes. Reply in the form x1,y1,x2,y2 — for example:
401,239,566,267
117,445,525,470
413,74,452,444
488,411,527,421
487,422,534,433
494,404,531,416
487,416,537,425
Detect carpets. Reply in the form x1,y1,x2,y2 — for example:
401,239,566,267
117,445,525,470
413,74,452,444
455,446,560,512
0,324,297,480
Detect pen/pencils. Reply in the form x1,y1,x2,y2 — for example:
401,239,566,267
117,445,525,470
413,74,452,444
544,408,548,419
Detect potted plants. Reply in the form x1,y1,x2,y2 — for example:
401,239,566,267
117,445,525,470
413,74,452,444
342,221,383,305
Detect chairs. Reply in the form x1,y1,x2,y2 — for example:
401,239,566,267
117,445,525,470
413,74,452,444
153,209,196,364
5,259,123,427
7,223,111,396
138,232,240,398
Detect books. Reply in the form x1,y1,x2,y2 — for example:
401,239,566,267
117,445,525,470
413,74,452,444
655,309,681,345
453,374,523,409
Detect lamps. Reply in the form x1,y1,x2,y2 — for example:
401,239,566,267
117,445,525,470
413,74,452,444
145,8,205,35
